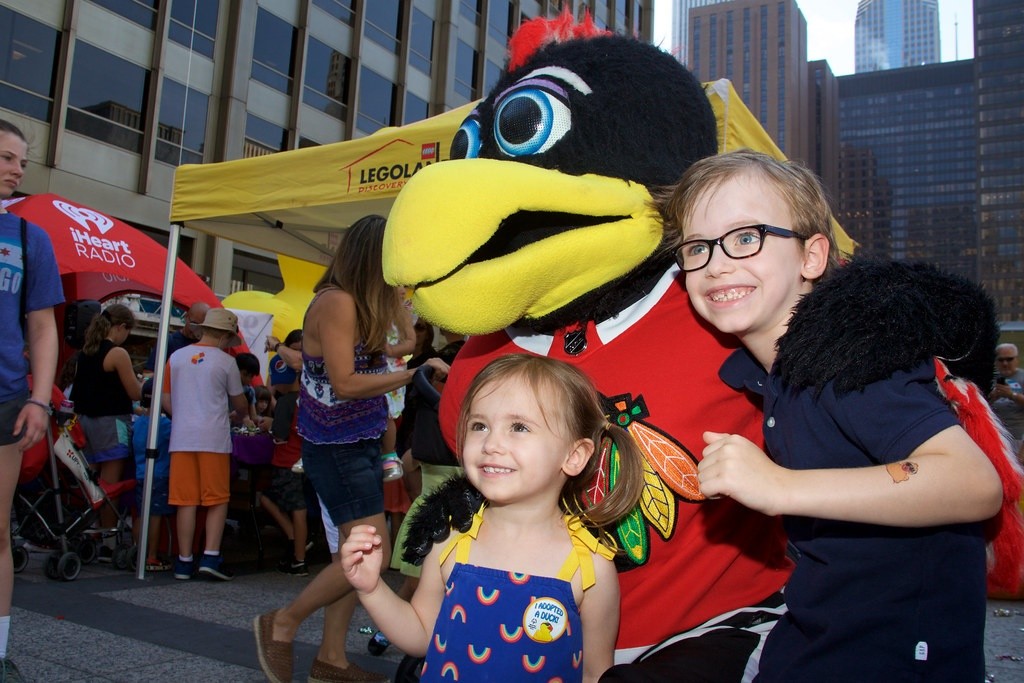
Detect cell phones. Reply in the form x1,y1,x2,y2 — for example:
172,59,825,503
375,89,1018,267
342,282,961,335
996,377,1005,385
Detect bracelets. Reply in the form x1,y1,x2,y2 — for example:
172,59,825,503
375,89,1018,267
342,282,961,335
274,343,285,353
1010,391,1017,401
25,400,53,416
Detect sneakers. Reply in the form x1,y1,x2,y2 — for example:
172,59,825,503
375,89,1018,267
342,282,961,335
174,557,195,579
278,560,309,577
199,554,234,581
23,540,63,552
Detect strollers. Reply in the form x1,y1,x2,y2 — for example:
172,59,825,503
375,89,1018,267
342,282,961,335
10,373,138,583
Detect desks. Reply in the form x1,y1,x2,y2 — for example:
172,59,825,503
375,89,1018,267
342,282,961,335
228,433,278,560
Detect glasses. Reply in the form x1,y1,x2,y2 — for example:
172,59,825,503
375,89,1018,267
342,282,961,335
416,326,427,332
188,314,198,324
996,356,1017,362
673,224,809,273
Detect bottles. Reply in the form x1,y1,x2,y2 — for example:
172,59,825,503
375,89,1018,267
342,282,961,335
56,399,74,426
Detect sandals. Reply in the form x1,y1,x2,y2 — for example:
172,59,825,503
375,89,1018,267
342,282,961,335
146,559,172,571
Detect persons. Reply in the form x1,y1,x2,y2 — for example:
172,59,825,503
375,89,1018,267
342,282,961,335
987,343,1024,455
0,118,66,683
252,213,451,683
141,302,210,389
132,377,172,572
257,355,309,579
644,147,1005,683
341,352,643,683
161,308,249,581
222,286,466,574
73,304,154,562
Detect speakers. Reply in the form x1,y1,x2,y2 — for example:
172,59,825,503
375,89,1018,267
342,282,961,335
64,299,102,349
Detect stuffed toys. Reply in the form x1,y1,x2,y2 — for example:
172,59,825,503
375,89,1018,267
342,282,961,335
380,5,1024,683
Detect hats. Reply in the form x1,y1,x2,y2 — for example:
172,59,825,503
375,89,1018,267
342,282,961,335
269,354,297,386
141,378,153,408
189,308,242,346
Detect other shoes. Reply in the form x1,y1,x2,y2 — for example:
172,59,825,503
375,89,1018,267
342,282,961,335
308,657,391,683
292,458,304,473
382,457,403,482
254,611,294,683
368,630,391,655
98,546,113,562
0,656,26,683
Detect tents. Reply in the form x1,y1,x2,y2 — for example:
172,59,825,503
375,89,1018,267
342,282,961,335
137,74,856,584
